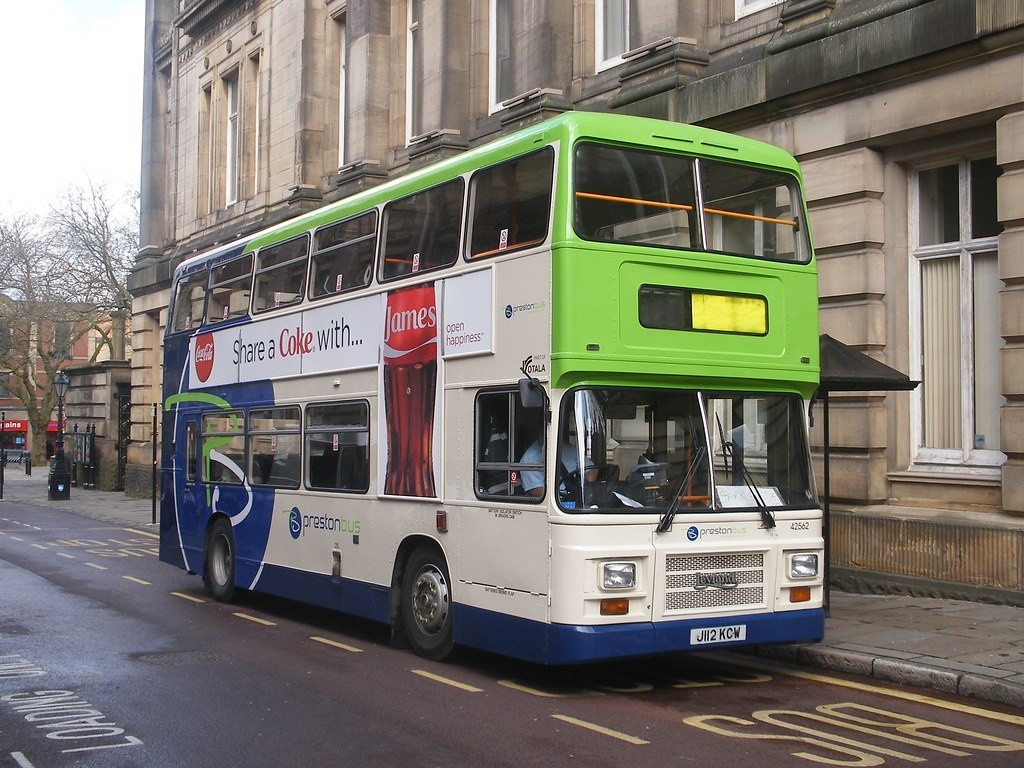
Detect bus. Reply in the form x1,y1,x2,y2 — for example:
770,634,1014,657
159,109,827,666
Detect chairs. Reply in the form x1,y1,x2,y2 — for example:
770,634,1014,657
210,438,529,497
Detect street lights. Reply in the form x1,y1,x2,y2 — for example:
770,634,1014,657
49,368,71,500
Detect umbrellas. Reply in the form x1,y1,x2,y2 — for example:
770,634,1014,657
818,334,920,614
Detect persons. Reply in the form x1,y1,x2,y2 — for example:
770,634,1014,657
520,417,599,497
0,451,7,501
